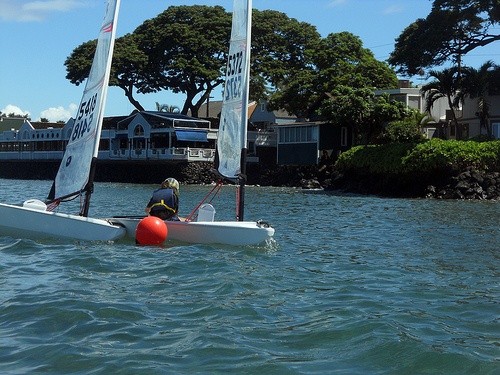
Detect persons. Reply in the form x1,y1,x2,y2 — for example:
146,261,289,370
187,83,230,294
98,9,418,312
145,177,187,221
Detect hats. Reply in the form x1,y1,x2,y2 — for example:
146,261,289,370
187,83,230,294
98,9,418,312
162,178,180,191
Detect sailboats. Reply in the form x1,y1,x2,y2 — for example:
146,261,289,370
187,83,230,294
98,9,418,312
0,0,128,244
104,0,276,247
